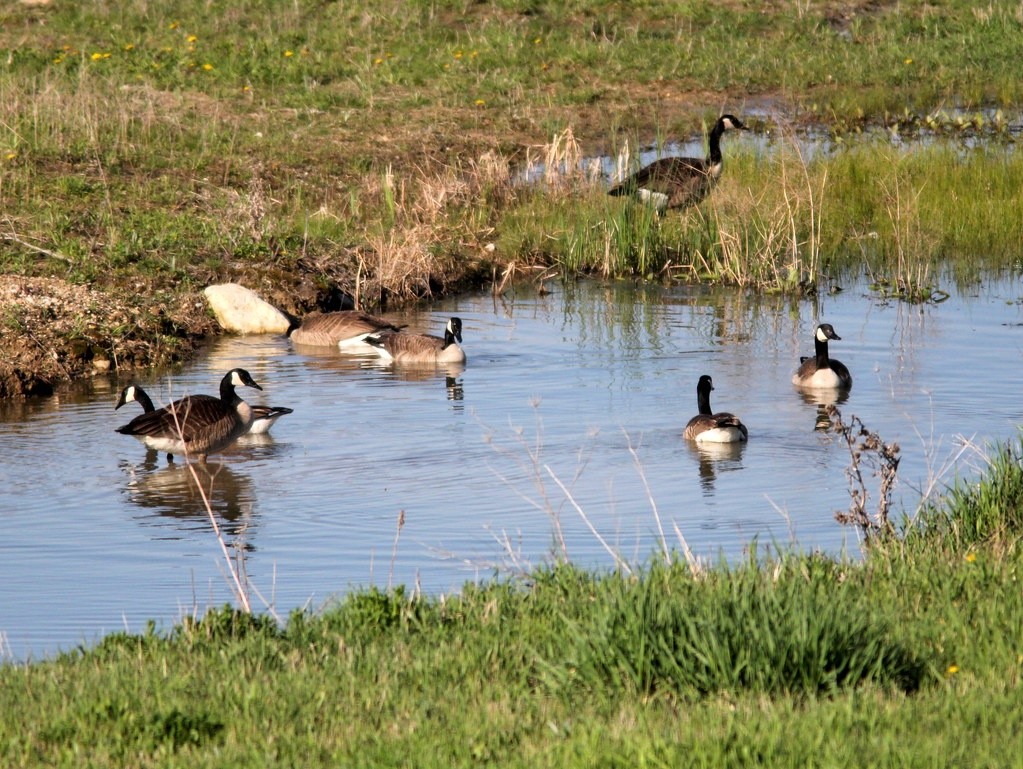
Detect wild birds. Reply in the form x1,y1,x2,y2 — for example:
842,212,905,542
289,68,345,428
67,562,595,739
114,368,264,470
115,383,294,434
288,300,410,348
792,324,853,389
683,374,748,443
362,316,467,364
606,114,752,222
